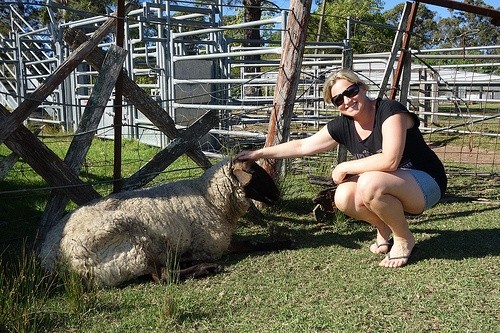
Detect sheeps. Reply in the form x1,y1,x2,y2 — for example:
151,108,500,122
40,143,281,289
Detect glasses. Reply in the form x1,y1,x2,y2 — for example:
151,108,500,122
331,83,361,107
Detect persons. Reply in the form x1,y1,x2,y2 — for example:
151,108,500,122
234,68,448,269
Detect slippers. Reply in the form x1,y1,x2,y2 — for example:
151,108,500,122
387,247,416,267
369,240,392,253
